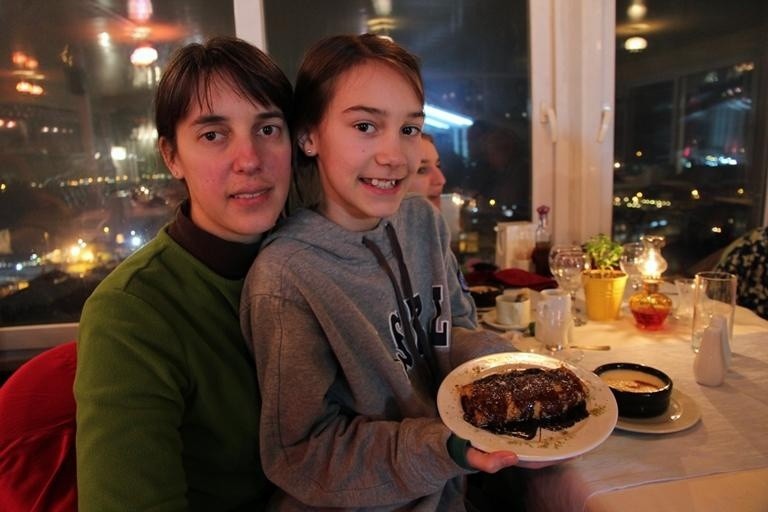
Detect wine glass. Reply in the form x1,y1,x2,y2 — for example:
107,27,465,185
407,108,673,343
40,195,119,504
469,242,588,345
623,243,645,294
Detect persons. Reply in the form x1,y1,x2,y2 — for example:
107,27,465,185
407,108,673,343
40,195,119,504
73,35,302,510
401,130,445,216
239,32,583,510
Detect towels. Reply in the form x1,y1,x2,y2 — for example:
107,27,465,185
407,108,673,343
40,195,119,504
492,266,559,293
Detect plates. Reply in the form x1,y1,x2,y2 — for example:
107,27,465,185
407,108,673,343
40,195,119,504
610,389,703,436
435,351,631,459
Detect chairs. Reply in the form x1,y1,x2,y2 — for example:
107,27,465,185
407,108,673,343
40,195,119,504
1,340,77,512
705,225,767,321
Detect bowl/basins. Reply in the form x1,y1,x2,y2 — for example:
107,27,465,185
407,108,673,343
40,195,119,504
592,363,683,413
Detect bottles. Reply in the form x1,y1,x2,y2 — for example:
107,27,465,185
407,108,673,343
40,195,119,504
694,326,725,386
708,312,732,369
630,282,671,332
534,206,552,250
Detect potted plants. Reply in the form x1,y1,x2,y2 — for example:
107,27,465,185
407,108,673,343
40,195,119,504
577,232,633,322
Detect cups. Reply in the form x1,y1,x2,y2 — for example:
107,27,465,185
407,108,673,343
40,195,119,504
692,275,737,355
675,276,689,322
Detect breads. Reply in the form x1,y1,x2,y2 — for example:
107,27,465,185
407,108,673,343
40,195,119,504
461,366,590,428
